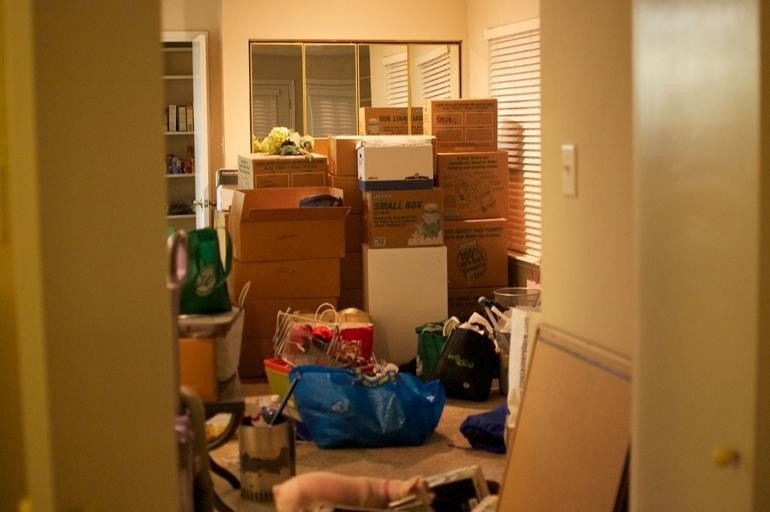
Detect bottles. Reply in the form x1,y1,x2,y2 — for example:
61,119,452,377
166,143,194,174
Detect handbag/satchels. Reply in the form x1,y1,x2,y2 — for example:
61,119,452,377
181,226,232,315
178,280,251,382
272,302,447,450
415,321,448,382
428,323,496,402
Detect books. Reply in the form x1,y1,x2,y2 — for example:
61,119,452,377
168,104,177,133
175,106,187,132
186,103,194,132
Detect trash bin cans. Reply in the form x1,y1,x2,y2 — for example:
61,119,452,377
494,328,510,396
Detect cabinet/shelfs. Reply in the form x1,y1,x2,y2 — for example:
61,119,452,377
163,31,207,239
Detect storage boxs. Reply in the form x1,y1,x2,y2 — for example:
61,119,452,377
214,97,509,449
